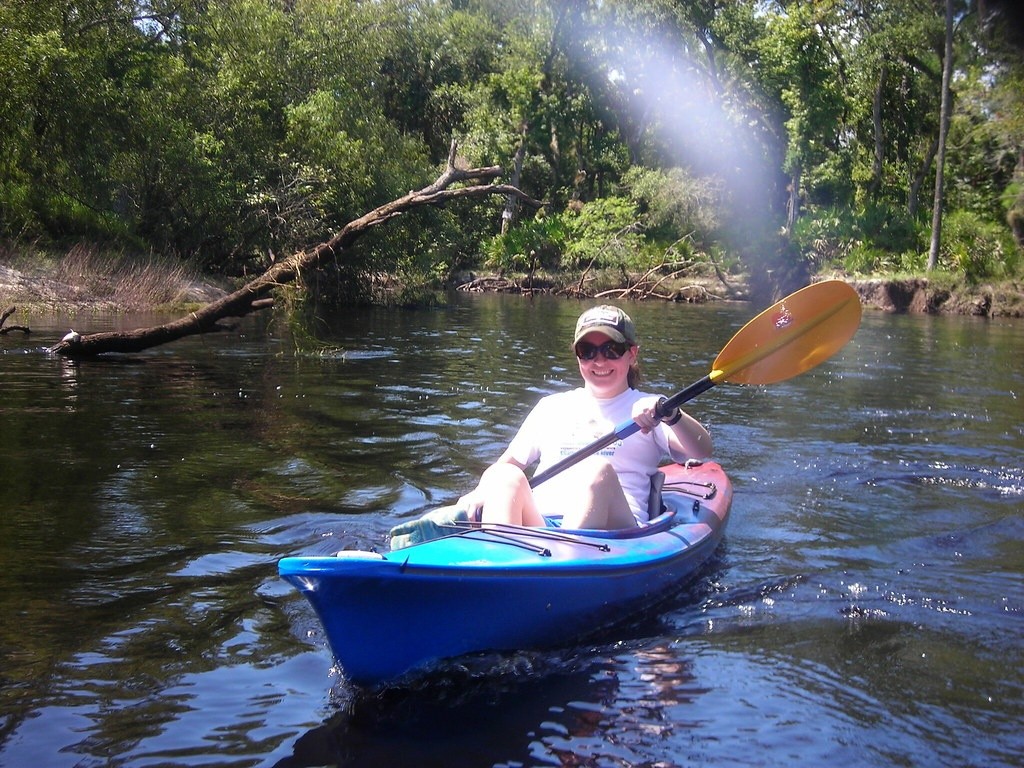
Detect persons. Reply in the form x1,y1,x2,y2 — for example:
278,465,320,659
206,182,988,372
456,301,712,531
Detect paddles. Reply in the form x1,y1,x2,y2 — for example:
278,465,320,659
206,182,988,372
469,280,862,525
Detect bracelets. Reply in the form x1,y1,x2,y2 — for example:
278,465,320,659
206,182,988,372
660,406,683,427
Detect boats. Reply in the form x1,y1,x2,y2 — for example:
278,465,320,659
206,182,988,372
273,457,732,687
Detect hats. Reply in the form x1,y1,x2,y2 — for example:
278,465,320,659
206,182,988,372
573,304,635,348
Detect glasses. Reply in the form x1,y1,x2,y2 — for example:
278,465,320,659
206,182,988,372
575,341,631,359
